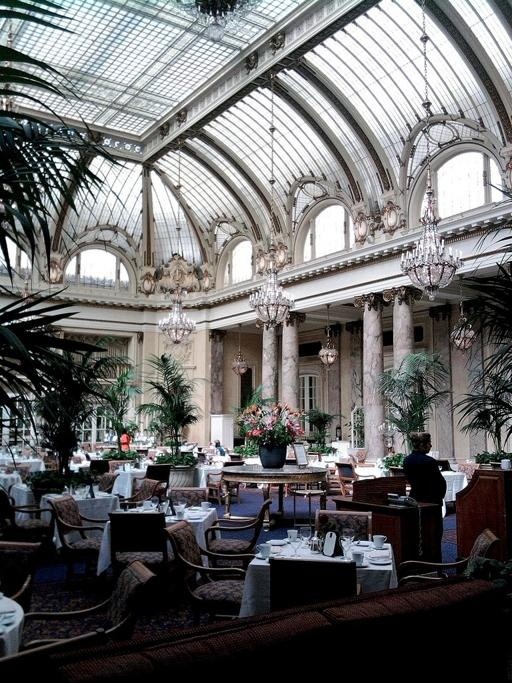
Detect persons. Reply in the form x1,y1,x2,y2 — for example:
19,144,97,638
214,439,225,456
403,431,447,562
120,429,130,453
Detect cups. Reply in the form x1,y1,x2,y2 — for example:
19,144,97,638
287,530,299,542
501,459,512,470
373,535,388,548
176,511,184,520
201,502,212,512
255,544,270,559
143,501,152,511
350,552,364,566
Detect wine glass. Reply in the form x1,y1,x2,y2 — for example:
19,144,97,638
290,540,302,558
339,535,351,559
344,529,354,552
300,527,312,550
174,506,185,521
151,497,159,513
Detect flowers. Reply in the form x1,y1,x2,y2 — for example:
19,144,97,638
234,398,306,449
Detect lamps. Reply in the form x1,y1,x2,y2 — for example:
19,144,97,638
156,137,197,347
244,72,297,333
448,276,478,353
396,0,464,301
231,322,249,377
317,305,339,370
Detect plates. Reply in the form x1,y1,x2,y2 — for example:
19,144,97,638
265,539,289,545
187,516,203,520
369,559,393,565
354,542,370,547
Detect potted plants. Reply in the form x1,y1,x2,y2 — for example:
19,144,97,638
134,352,204,488
